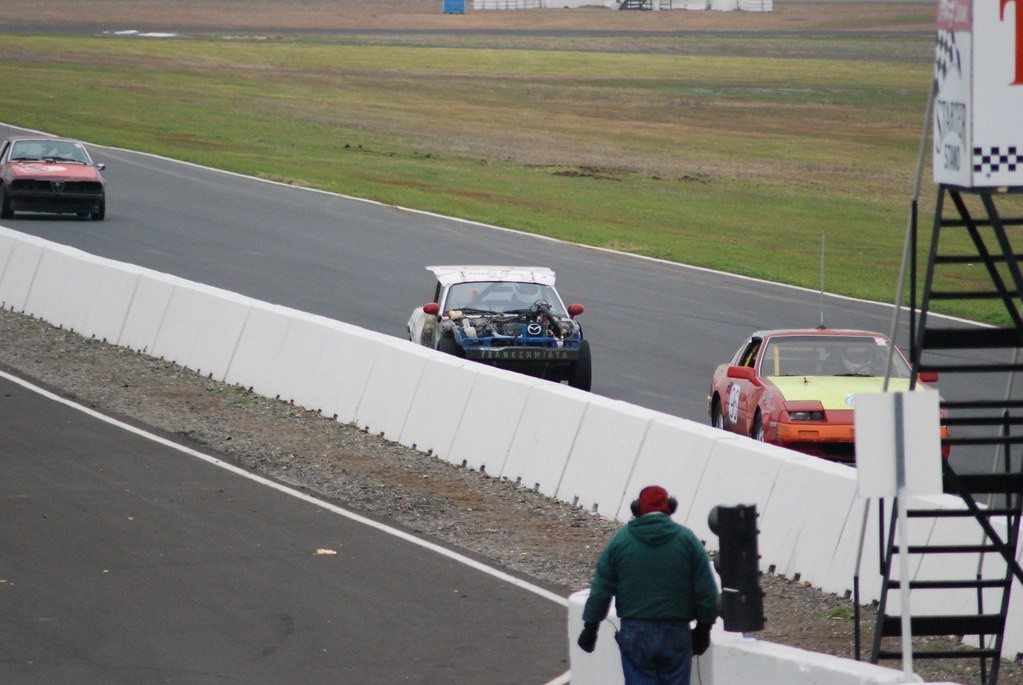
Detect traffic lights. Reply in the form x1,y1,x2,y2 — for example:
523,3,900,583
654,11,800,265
707,506,767,633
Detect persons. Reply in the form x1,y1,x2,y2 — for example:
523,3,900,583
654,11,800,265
827,340,889,376
576,485,718,685
49,142,74,159
509,282,550,310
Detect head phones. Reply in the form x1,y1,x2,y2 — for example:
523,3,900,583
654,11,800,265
630,494,678,517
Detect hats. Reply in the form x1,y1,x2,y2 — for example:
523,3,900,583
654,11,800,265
639,486,672,515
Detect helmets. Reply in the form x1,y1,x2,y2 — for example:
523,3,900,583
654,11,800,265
56,141,74,159
514,283,540,304
841,345,872,373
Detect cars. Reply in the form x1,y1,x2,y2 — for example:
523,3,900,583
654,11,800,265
0,136,108,224
707,329,951,467
407,264,592,395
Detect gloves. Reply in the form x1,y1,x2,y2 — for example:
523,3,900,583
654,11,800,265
692,623,711,656
576,622,598,653
